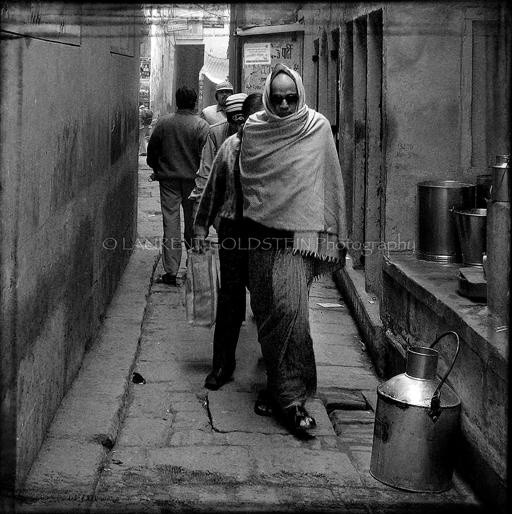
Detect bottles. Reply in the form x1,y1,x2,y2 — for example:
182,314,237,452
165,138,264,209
489,154,511,203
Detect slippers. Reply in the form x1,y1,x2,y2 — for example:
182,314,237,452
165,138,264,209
278,403,317,433
253,386,279,418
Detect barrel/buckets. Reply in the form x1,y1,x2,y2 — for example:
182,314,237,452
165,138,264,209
368,331,461,493
448,207,488,269
416,179,476,264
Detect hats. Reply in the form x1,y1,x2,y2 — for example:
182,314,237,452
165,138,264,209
216,81,234,92
224,92,249,113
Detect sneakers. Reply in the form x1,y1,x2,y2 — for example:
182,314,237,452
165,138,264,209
202,362,237,390
154,274,177,287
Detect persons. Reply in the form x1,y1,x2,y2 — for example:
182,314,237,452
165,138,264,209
193,94,268,391
139,101,151,155
146,86,209,285
239,62,346,430
201,81,234,124
187,92,247,238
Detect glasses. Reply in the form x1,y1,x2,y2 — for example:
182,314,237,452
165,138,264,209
270,94,298,105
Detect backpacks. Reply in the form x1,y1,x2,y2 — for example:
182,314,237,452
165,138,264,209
142,110,153,125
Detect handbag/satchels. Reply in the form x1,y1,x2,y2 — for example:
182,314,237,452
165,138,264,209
184,245,221,330
